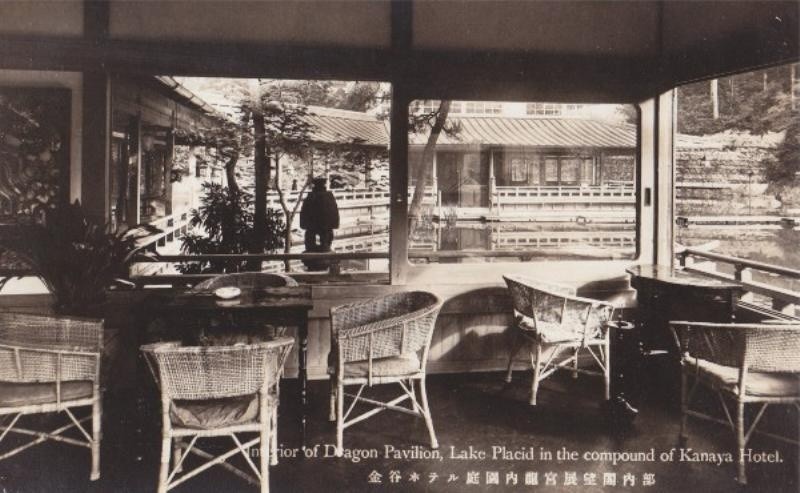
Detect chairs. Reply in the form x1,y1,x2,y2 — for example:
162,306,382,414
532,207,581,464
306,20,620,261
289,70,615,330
500,270,615,409
139,335,297,493
187,270,296,292
0,304,120,488
664,317,799,487
326,289,443,458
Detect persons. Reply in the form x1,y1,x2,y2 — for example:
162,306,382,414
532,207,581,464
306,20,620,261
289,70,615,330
300,175,339,251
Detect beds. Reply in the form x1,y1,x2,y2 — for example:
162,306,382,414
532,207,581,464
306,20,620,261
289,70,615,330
132,287,316,464
